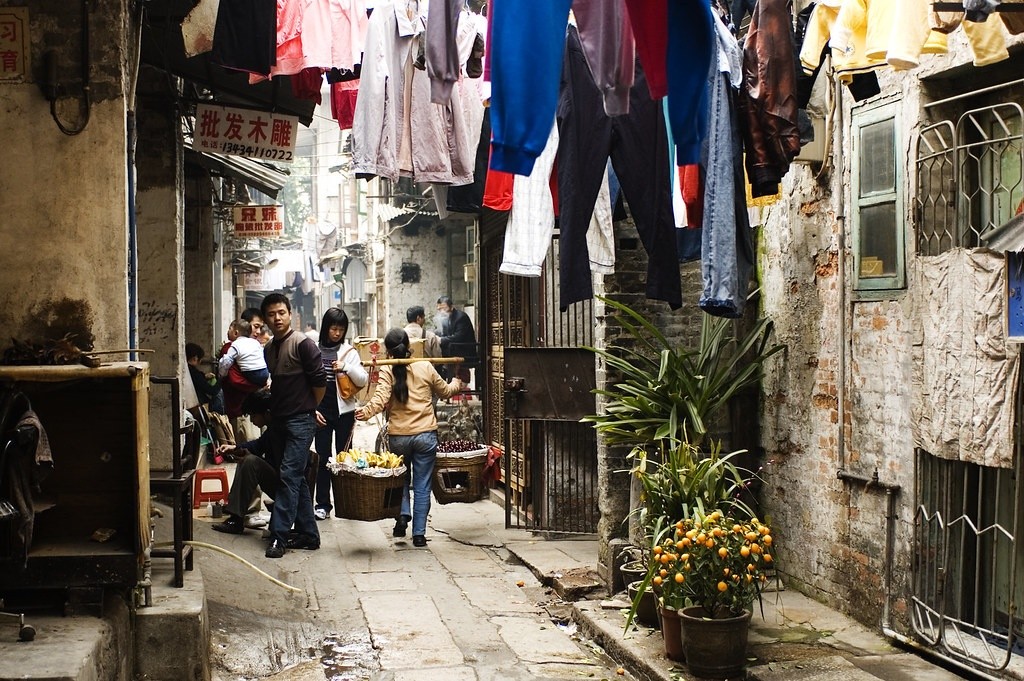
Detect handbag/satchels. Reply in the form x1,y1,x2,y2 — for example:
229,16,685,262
335,347,367,401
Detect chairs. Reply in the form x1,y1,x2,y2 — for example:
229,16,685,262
0,391,54,641
146,373,198,588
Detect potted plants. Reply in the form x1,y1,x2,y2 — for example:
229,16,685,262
577,282,787,681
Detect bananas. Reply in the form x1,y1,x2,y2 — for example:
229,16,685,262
336,446,404,470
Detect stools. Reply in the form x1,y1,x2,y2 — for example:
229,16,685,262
193,469,229,508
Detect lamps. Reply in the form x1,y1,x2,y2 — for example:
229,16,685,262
226,254,278,270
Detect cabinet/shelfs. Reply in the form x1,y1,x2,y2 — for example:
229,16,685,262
0,360,154,618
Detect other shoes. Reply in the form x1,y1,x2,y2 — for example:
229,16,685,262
243,511,271,528
314,508,330,519
392,515,408,537
211,518,245,534
265,533,286,558
413,535,426,547
284,534,320,551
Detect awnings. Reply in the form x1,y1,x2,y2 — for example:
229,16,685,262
328,162,349,179
183,137,291,200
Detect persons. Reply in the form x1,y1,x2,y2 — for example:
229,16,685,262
314,307,369,520
260,293,327,559
303,320,320,343
355,328,463,546
258,328,269,344
403,296,477,412
186,307,287,533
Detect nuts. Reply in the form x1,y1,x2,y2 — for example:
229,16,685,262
436,439,482,452
